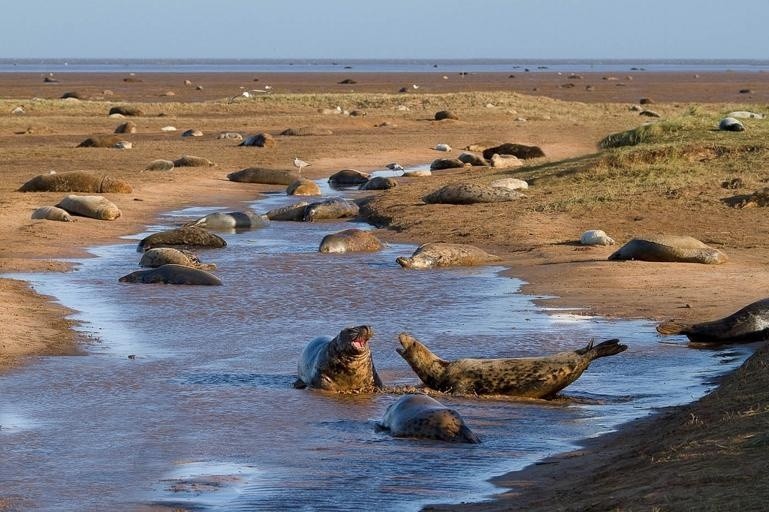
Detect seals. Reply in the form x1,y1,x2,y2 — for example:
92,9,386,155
368,391,482,447
226,166,300,186
317,104,460,127
395,329,628,401
15,170,133,194
327,168,369,184
287,62,760,100
30,205,72,222
218,125,334,148
718,111,766,132
10,72,215,173
183,211,270,232
428,139,546,170
629,97,660,118
118,263,223,286
358,175,398,191
607,230,727,266
656,297,769,344
286,176,321,196
137,227,227,250
290,324,384,396
139,247,217,273
420,179,527,203
483,101,552,123
395,242,502,271
318,228,383,253
581,229,614,246
55,194,122,222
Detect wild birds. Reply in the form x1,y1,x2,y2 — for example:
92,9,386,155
293,157,311,174
385,161,405,177
